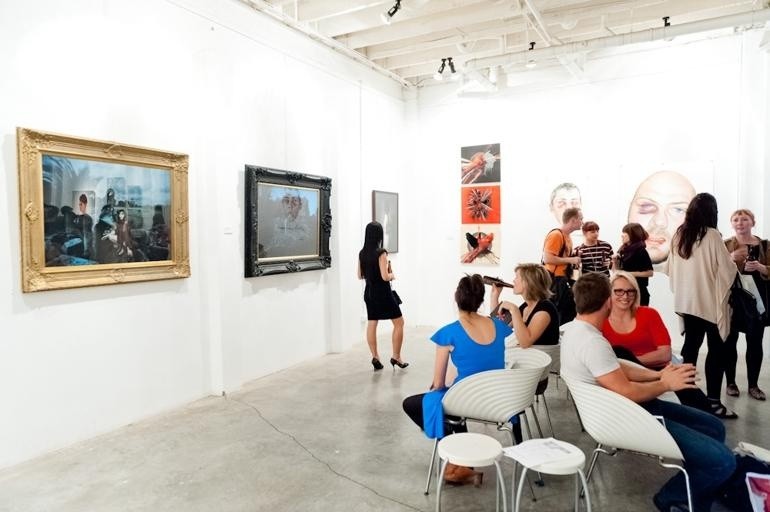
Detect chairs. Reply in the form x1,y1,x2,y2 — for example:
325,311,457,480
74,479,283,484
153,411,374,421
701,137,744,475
421,343,550,505
507,338,565,436
565,356,699,509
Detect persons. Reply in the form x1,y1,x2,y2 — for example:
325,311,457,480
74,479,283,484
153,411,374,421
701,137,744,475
274,194,310,246
549,183,581,225
74,194,165,263
628,171,697,264
357,221,409,370
402,275,518,487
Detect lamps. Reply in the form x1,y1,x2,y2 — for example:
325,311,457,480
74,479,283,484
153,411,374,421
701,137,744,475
662,15,671,33
381,1,402,25
432,58,447,80
526,59,536,68
526,42,537,53
447,57,458,76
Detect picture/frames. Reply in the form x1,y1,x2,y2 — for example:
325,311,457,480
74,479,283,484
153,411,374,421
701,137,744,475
241,163,334,279
12,125,192,296
370,188,401,254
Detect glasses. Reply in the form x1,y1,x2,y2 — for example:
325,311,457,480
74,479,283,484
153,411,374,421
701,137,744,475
614,288,637,297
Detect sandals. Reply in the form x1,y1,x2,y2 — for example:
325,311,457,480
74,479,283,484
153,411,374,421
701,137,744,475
707,396,738,420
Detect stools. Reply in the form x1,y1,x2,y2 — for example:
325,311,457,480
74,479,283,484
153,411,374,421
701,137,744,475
431,431,513,511
513,437,596,509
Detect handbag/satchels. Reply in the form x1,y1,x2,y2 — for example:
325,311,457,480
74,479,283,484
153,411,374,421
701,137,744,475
388,279,404,307
729,269,762,336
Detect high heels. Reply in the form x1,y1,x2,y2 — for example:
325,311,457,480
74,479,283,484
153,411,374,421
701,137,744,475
372,357,383,370
390,357,409,370
436,462,484,488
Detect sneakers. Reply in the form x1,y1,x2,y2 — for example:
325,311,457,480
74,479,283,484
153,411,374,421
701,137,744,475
748,385,767,401
726,384,740,396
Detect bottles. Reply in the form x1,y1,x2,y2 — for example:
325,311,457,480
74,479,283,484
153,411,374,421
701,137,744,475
573,248,579,269
482,276,514,289
388,260,392,281
744,246,755,263
601,250,606,264
